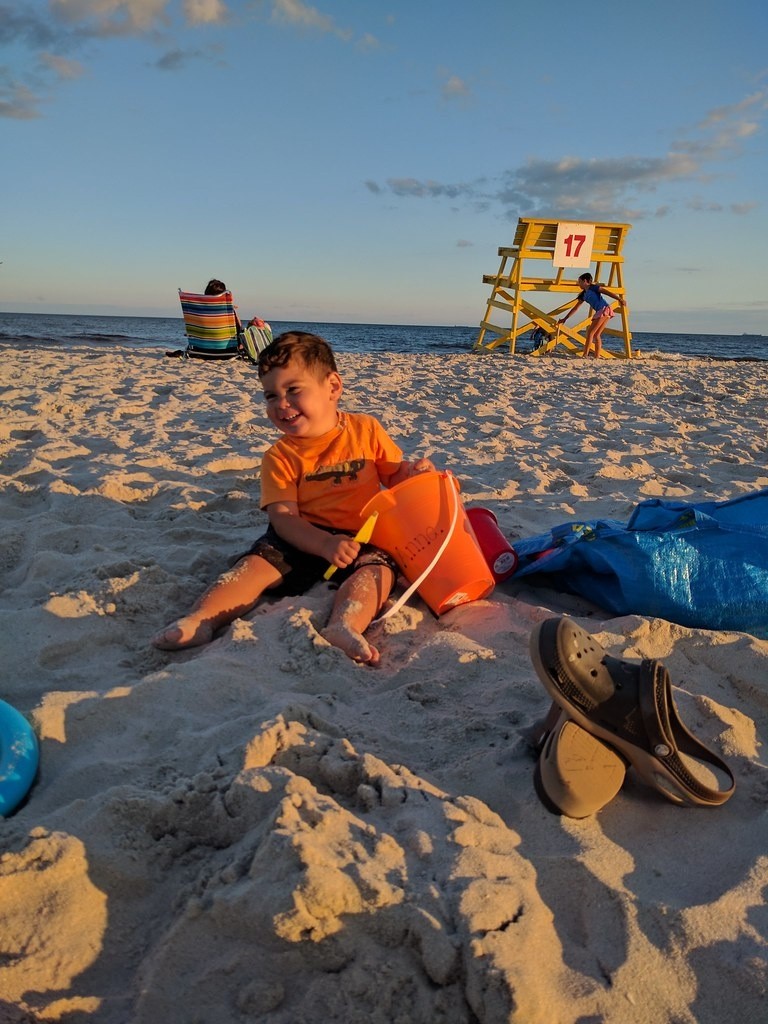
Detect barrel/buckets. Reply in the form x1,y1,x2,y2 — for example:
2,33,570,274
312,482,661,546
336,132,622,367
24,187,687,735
358,472,519,616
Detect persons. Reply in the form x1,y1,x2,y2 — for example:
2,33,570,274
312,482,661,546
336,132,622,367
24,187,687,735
205,280,242,326
155,330,435,662
558,273,626,359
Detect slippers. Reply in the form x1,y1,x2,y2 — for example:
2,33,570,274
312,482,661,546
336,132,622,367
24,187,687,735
530,617,736,809
527,700,629,820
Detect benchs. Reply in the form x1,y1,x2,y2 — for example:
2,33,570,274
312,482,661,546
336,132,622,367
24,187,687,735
473,217,635,359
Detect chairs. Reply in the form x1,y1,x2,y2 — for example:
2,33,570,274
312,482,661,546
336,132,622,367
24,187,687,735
178,287,273,361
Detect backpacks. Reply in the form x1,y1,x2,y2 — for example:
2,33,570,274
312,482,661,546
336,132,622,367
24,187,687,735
534,327,551,347
